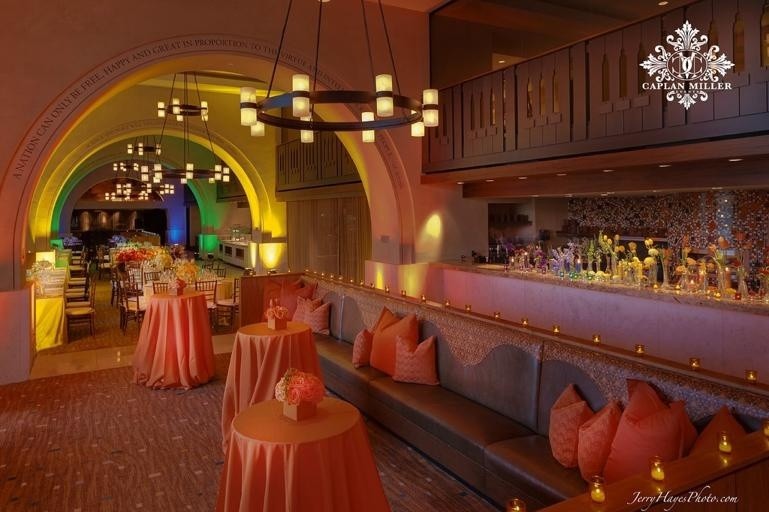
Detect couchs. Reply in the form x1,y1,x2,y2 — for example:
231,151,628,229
239,269,768,512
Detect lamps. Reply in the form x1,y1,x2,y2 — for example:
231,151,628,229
100,135,176,204
141,71,231,185
239,0,441,143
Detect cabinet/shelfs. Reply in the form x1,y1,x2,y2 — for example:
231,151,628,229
437,267,768,384
285,197,373,282
219,241,257,270
418,1,769,173
215,158,249,203
274,130,366,190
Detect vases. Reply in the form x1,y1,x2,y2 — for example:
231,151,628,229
268,317,287,329
168,287,183,296
283,401,317,420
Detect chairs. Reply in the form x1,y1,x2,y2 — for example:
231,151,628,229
216,278,240,331
151,281,169,294
65,275,98,343
201,262,214,270
65,263,86,301
67,244,93,293
144,272,162,285
66,271,97,334
108,261,148,335
194,279,218,329
209,267,227,277
64,264,92,304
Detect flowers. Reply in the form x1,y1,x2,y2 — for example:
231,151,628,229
264,306,289,329
275,368,326,420
167,277,187,296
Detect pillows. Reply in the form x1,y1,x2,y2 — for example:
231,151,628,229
392,332,440,386
304,297,332,336
549,383,595,469
603,378,700,490
292,295,323,322
689,404,748,455
370,305,419,376
577,399,625,484
352,328,374,369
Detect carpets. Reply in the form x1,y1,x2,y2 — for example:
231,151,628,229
0,352,466,510
36,273,238,355
